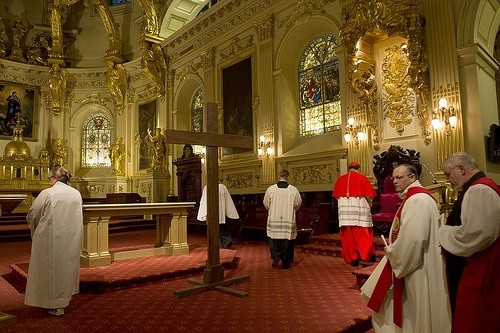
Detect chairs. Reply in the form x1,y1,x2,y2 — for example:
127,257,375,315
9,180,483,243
369,145,422,235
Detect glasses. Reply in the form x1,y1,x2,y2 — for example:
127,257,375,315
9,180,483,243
389,174,412,182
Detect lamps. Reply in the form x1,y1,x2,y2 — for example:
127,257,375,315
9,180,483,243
431,98,457,136
199,148,205,164
343,117,365,148
258,136,271,157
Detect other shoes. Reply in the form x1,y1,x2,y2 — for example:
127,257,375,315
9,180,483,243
47,309,65,317
272,256,280,268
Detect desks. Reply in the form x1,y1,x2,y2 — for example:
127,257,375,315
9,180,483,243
80,202,196,268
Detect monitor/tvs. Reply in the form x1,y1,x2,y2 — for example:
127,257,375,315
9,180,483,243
490,124,500,161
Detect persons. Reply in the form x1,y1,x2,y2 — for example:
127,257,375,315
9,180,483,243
263,170,302,269
24,167,83,317
360,152,500,333
52,138,65,167
10,15,26,47
104,62,126,109
110,137,125,175
197,183,240,248
148,128,165,171
140,41,168,97
48,64,66,112
331,161,378,266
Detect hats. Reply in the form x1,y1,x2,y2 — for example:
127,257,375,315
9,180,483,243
349,160,360,167
